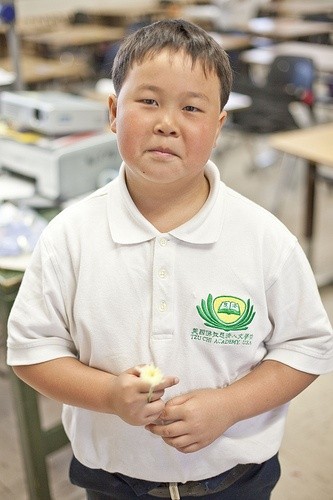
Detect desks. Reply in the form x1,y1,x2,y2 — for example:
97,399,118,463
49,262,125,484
0,0,333,288
0,207,71,500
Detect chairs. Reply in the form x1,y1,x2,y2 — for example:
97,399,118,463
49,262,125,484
231,55,315,130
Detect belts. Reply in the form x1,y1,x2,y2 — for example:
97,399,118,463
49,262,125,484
147,463,256,499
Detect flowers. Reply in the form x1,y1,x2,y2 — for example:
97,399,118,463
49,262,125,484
135,362,165,402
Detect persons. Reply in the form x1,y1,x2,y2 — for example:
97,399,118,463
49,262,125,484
6,16,333,500
